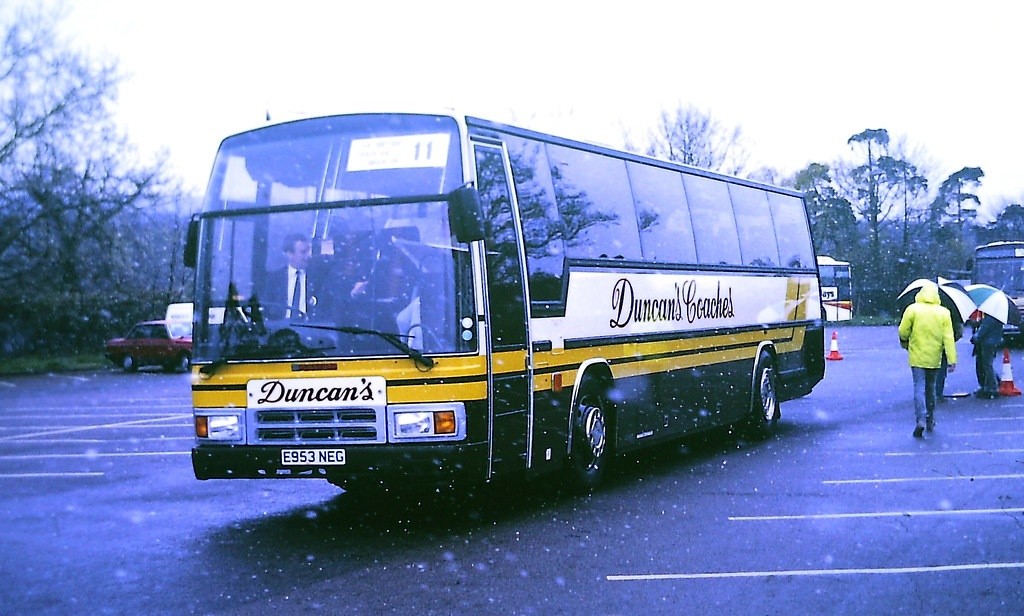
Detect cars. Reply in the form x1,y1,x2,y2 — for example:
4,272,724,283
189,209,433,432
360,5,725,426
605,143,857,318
104,320,196,372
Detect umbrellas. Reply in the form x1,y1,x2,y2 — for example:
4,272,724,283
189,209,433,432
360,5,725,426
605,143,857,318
895,274,977,330
964,284,1022,327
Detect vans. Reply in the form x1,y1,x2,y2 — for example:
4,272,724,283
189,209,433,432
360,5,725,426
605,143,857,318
166,302,251,343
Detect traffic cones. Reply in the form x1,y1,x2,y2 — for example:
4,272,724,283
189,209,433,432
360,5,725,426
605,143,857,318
826,331,843,360
997,348,1021,395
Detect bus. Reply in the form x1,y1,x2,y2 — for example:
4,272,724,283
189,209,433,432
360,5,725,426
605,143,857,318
965,240,1024,344
179,111,828,509
810,254,854,324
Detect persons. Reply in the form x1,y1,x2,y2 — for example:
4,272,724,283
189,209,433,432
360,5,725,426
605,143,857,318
898,283,958,438
250,231,345,329
969,312,1003,401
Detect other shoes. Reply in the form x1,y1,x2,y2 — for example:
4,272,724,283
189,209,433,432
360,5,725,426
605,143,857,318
912,423,924,439
928,422,936,432
